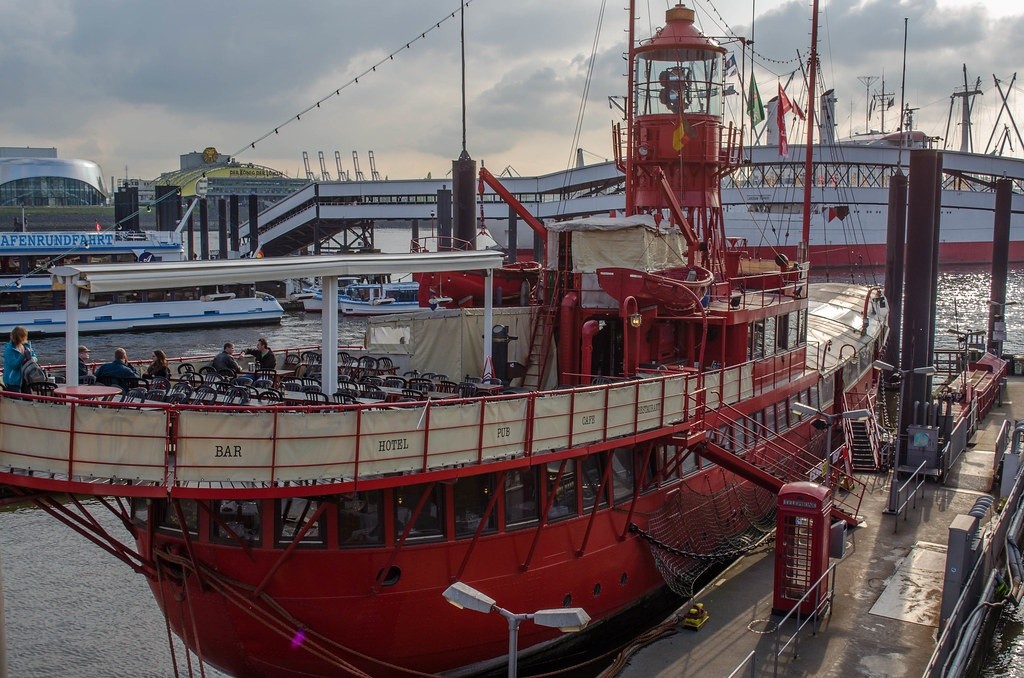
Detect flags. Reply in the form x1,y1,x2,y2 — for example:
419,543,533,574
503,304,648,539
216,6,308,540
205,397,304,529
868,97,894,121
673,51,806,158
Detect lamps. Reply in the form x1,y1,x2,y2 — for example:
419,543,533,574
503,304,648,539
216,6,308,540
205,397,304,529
428,297,453,310
630,313,642,328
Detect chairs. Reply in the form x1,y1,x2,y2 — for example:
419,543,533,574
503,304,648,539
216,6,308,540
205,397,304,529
28,351,612,413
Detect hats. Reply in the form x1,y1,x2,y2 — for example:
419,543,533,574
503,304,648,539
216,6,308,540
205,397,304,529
78,345,91,352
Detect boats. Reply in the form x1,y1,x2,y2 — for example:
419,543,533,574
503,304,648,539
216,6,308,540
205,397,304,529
1,2,1023,678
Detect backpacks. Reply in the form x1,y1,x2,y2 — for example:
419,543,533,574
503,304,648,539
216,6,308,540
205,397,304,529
19,350,47,390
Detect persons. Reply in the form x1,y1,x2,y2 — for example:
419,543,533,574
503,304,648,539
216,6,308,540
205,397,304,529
212,341,242,383
2,326,37,402
78,343,169,389
239,338,277,371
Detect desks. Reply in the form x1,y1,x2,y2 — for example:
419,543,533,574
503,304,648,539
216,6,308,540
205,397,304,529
42,384,174,410
164,373,226,383
237,369,293,376
378,375,503,395
210,383,384,405
287,358,399,372
284,378,459,402
151,389,286,406
136,378,163,384
